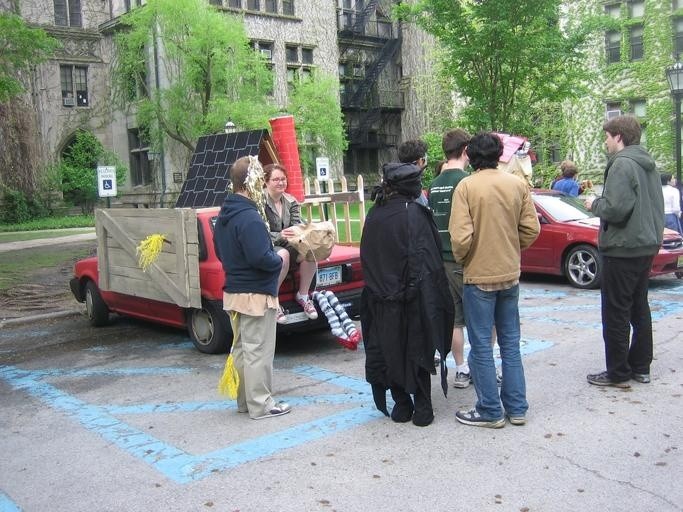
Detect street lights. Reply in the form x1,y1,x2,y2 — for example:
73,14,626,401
223,113,237,134
665,55,683,213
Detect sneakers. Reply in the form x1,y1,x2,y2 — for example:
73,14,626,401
295,294,318,320
634,372,650,383
455,368,527,428
587,371,632,388
253,401,291,419
276,305,287,322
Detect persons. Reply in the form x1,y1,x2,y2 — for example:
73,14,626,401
448,132,541,429
583,116,662,388
550,161,592,195
359,163,456,427
398,129,502,388
551,163,577,197
211,153,290,420
661,171,682,234
259,165,320,321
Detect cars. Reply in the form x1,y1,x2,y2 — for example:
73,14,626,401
520,187,682,289
68,207,366,353
